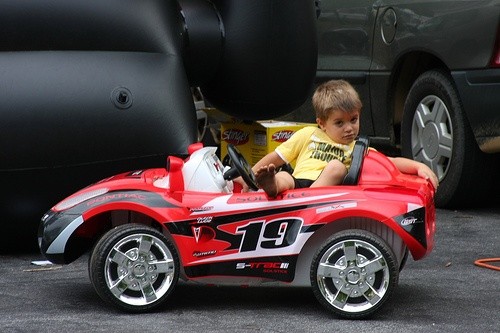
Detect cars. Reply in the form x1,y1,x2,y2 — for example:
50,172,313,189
271,0,500,210
37,137,437,320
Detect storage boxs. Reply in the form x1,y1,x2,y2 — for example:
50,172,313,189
204,120,319,169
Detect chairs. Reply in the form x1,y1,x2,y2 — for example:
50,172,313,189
341,136,370,186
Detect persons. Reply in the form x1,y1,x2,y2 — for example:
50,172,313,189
233,79,439,198
